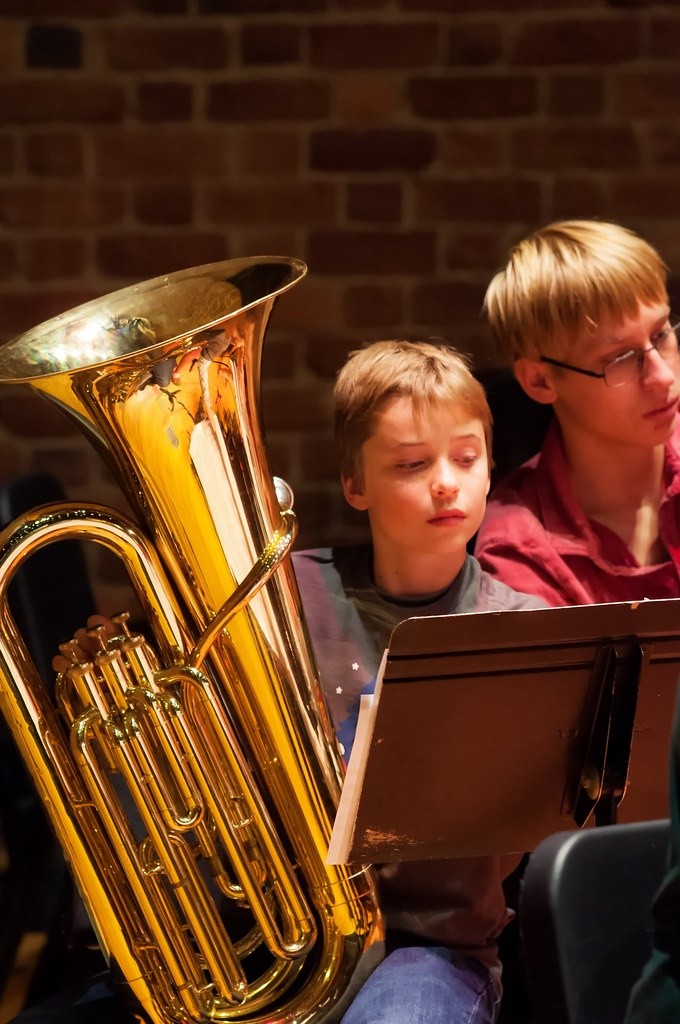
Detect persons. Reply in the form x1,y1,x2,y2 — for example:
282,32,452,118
50,334,561,1024
469,216,680,605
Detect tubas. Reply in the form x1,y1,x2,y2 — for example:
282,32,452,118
0,256,390,1024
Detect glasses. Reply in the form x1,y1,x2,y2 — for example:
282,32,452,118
541,327,678,388
